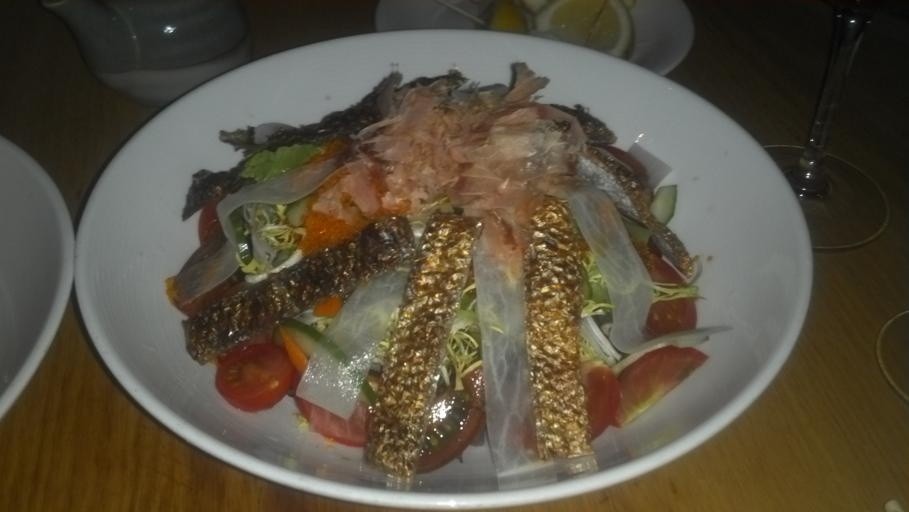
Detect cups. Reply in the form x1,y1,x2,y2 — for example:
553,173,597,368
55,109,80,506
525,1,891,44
41,0,253,111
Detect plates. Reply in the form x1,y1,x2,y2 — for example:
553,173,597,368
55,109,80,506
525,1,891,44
366,0,698,73
1,134,78,419
77,26,817,512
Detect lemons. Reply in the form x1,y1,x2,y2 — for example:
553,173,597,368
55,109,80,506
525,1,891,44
536,0,636,61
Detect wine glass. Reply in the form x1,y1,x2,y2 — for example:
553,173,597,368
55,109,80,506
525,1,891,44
759,0,892,249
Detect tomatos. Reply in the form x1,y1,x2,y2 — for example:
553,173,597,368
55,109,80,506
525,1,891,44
214,342,709,473
